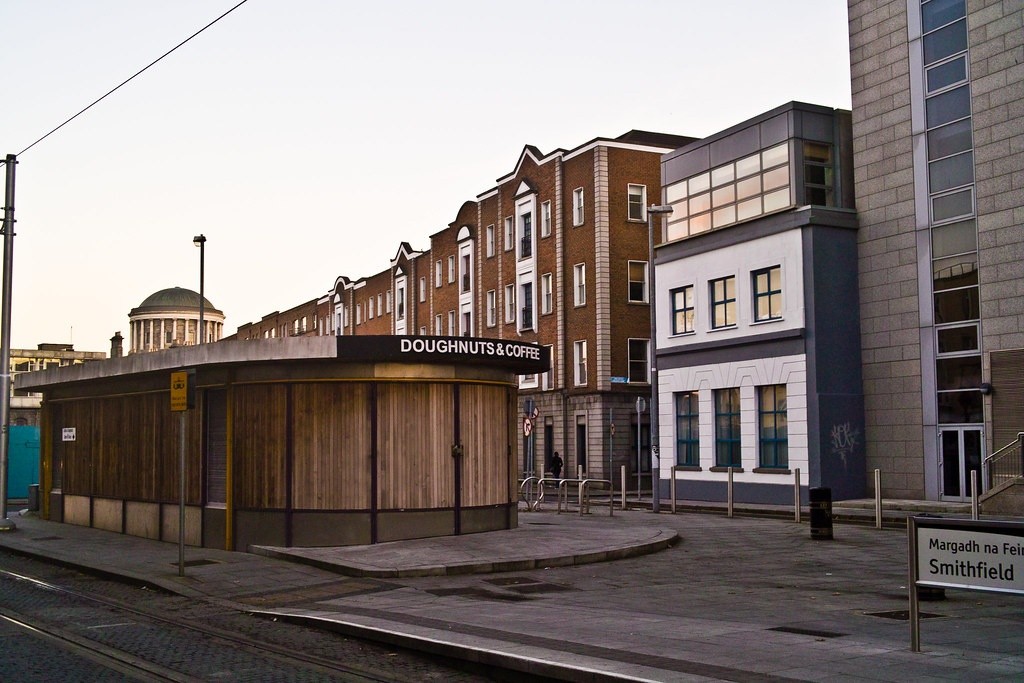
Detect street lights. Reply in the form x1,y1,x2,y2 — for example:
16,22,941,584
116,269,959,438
193,233,206,344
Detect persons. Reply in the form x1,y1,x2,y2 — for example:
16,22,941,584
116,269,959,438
549,452,563,490
